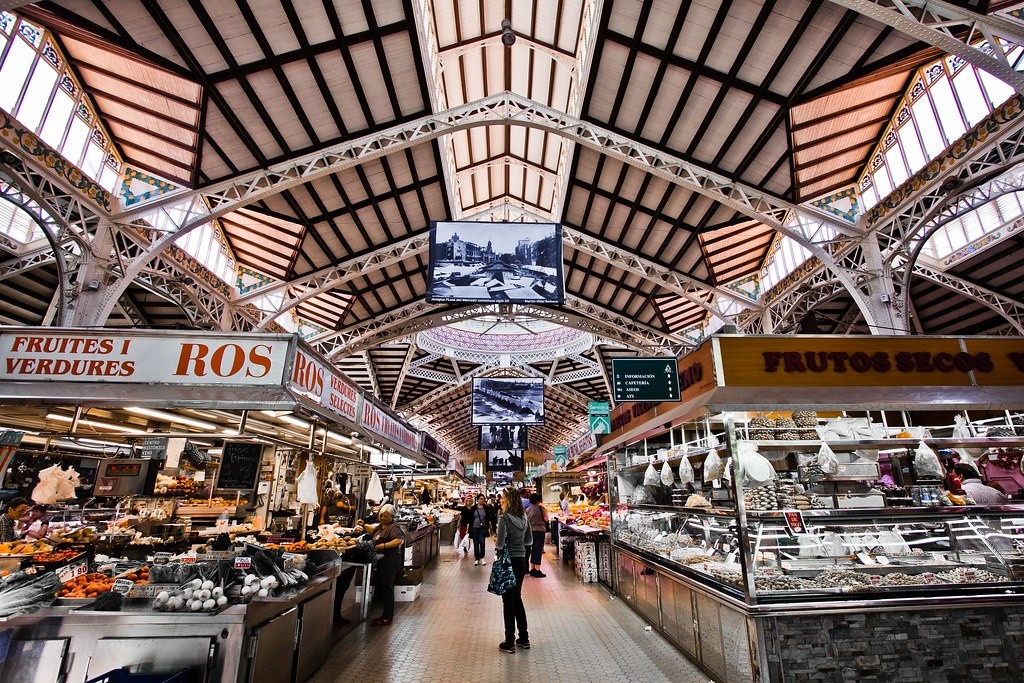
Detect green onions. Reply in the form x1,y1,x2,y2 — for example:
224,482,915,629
258,551,309,586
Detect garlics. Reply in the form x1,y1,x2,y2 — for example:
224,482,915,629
153,564,277,611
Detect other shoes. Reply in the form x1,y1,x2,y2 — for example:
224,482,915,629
516,637,530,649
530,569,537,576
534,570,546,578
474,560,479,566
499,641,516,653
525,571,530,574
481,558,486,565
372,616,392,625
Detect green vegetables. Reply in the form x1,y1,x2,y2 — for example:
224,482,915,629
0,569,64,616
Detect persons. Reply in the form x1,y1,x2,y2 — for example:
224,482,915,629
526,493,548,578
520,489,531,574
19,503,49,542
952,463,1013,552
491,486,531,654
320,491,351,524
357,504,405,624
422,484,432,507
441,492,447,502
489,426,526,449
559,493,570,516
0,497,29,543
474,492,503,537
469,493,492,565
456,497,473,554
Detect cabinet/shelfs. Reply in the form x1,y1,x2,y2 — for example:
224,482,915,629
0,566,340,683
405,525,441,571
441,516,458,542
44,509,131,555
604,404,1024,683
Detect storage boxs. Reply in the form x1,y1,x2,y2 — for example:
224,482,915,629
355,585,374,604
558,523,611,583
395,566,424,602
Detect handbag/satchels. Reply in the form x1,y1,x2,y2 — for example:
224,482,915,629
459,533,470,554
540,506,551,532
454,528,460,549
480,523,491,537
487,544,517,596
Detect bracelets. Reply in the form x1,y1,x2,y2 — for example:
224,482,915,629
383,543,385,549
